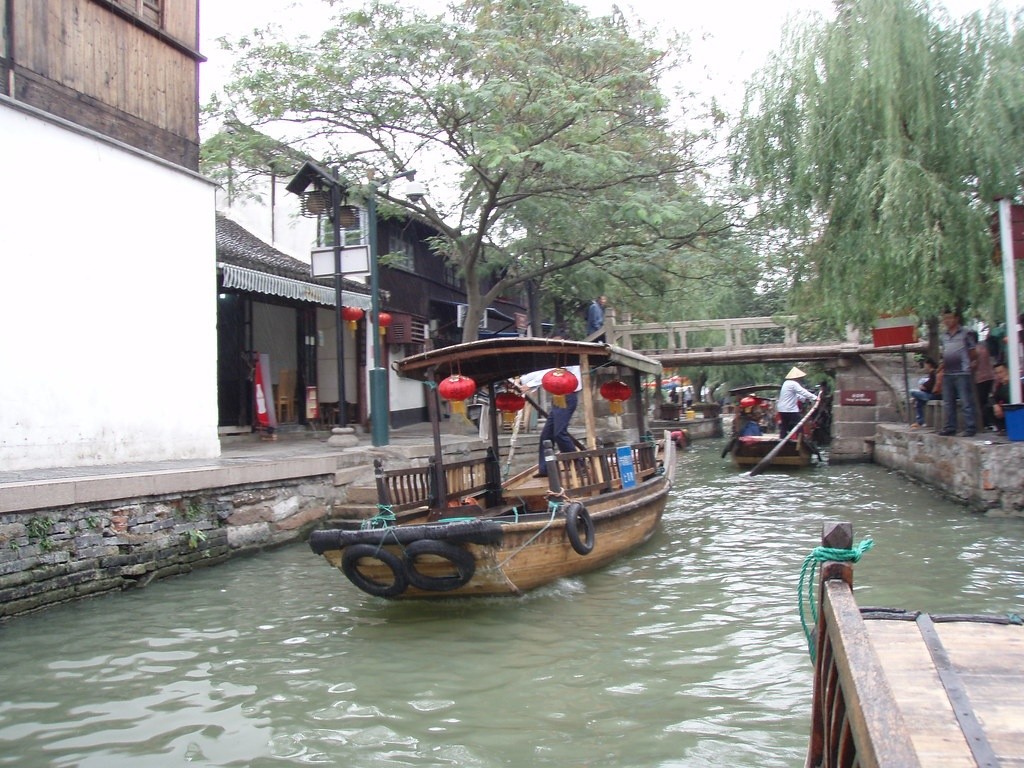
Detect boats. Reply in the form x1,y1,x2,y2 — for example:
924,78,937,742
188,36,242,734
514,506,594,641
648,381,725,443
306,336,689,601
721,383,821,468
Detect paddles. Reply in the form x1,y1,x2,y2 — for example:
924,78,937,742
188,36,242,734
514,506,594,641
749,389,824,477
505,378,592,462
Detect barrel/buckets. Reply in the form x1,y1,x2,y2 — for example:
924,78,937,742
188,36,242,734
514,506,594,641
1000,403,1024,441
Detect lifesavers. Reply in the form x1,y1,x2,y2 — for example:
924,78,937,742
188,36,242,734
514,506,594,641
721,439,735,459
564,502,595,556
402,539,476,593
341,543,408,598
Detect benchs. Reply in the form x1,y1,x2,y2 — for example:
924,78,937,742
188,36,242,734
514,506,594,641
924,399,966,431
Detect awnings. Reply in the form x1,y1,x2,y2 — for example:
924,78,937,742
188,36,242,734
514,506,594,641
217,261,373,311
433,298,517,338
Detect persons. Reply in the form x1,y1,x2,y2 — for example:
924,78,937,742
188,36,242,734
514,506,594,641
517,336,586,477
671,429,688,446
778,366,820,439
910,358,937,429
742,415,762,436
587,296,608,343
938,309,1024,436
669,386,693,412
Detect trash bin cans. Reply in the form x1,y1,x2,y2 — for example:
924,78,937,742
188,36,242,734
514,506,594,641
1001,403,1024,442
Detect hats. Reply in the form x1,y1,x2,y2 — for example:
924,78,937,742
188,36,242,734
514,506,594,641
785,367,807,379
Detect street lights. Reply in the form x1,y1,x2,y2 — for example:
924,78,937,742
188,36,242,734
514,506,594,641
363,164,427,446
284,162,352,428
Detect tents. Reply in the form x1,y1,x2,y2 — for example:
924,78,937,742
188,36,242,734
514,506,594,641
369,312,391,335
599,377,631,416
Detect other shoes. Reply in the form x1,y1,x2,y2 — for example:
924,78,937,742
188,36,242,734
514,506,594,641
939,428,956,436
958,431,975,437
534,470,549,480
911,422,922,428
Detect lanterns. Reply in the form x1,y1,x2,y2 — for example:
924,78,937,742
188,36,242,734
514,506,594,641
495,392,525,424
438,374,476,413
341,306,363,330
541,368,579,408
740,396,768,413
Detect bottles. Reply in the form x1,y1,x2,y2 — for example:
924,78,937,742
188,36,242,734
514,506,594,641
256,384,267,414
